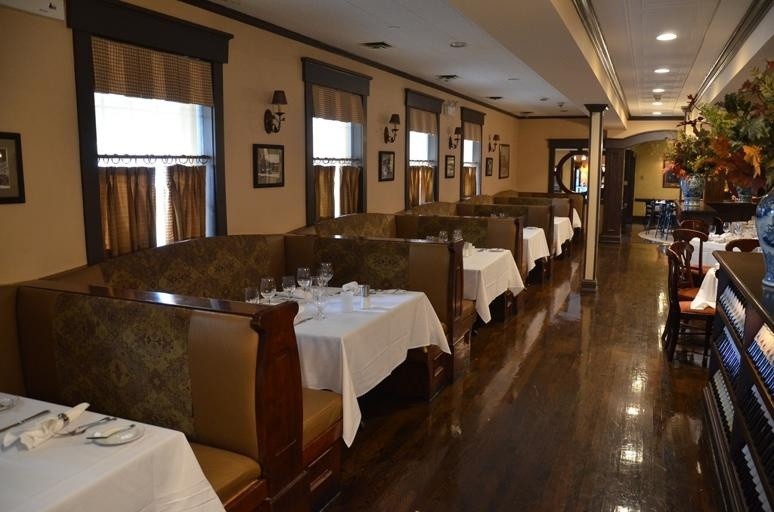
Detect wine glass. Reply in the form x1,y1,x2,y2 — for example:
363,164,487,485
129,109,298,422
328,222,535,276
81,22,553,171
241,262,334,319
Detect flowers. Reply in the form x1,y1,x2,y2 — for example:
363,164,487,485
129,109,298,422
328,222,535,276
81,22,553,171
662,57,774,198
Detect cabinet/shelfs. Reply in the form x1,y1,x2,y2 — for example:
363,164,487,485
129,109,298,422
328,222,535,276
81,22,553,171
691,250,774,510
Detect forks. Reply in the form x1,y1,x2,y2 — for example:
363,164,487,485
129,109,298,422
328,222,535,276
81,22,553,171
58,415,117,437
85,422,137,440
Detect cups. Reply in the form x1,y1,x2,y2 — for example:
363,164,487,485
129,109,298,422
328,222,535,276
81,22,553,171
708,221,758,242
424,230,463,240
339,291,353,313
463,242,472,257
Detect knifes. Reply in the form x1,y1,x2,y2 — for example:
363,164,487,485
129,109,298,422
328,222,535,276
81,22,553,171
0,408,49,431
292,317,311,326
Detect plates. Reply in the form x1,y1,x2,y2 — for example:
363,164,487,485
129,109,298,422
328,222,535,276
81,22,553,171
89,422,148,446
0,394,17,412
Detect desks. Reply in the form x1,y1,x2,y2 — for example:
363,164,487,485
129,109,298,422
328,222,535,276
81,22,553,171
0,390,227,511
242,285,453,446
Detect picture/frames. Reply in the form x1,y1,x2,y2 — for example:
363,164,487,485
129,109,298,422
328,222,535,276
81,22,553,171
0,131,29,207
252,144,286,187
498,143,510,179
378,150,396,182
485,157,493,177
444,154,456,179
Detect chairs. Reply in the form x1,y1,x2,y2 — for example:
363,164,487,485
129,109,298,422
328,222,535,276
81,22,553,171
630,171,773,369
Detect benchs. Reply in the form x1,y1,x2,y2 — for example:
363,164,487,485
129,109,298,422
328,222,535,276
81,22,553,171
17,233,458,510
290,188,589,362
0,283,282,508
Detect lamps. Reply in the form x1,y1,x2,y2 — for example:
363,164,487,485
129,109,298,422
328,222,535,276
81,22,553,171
264,89,287,134
384,113,400,145
448,126,464,152
487,133,500,154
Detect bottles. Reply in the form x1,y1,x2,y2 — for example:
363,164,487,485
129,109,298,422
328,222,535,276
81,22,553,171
358,284,372,310
